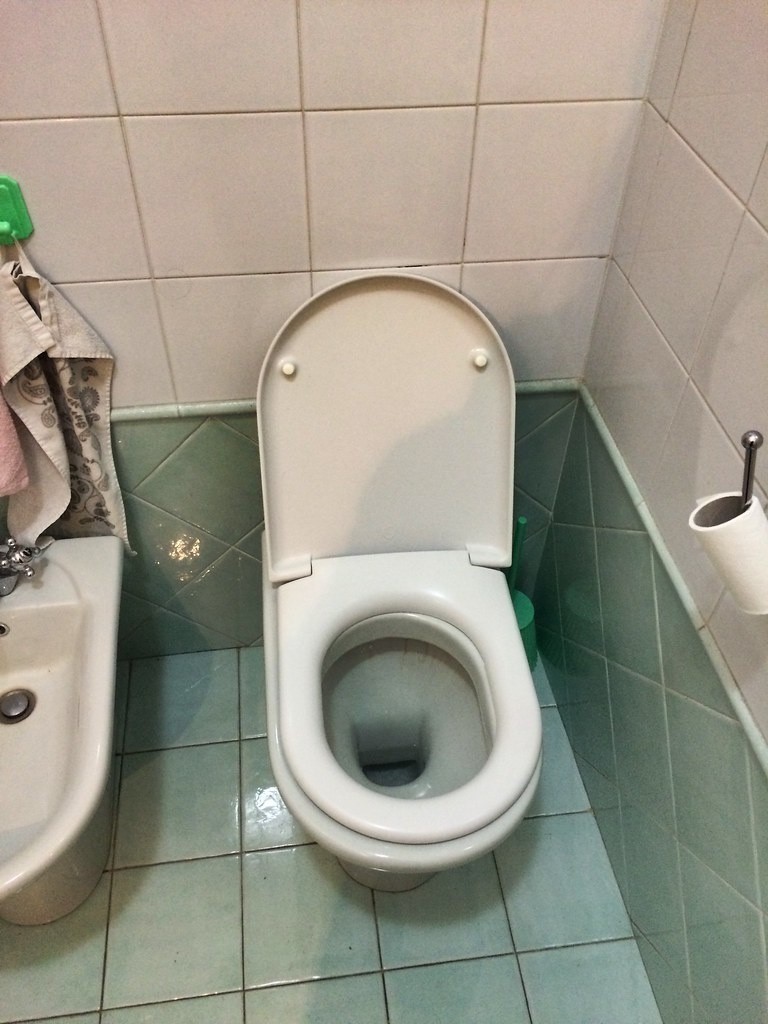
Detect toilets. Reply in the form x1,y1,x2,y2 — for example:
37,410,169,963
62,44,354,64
254,274,545,896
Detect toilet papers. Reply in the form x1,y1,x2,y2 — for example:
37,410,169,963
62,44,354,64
688,491,768,616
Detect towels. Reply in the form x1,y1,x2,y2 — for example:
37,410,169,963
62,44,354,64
1,269,138,559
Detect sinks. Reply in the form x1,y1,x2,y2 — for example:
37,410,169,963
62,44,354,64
0,534,127,928
